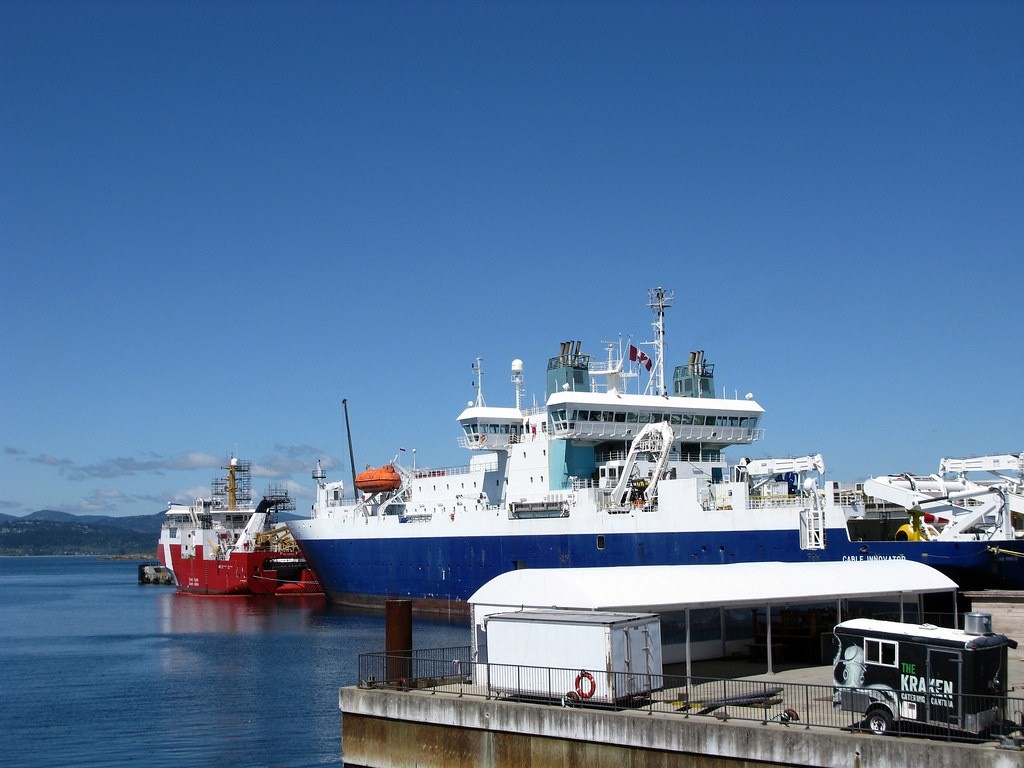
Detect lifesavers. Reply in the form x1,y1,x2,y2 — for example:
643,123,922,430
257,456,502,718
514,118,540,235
575,672,596,698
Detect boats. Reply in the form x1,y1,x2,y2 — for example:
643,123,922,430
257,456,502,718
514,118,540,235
155,443,323,594
352,466,400,490
138,560,172,585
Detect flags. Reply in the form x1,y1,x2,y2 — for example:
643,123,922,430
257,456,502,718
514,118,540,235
629,344,652,371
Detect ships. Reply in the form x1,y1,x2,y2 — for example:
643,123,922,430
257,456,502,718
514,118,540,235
283,284,1024,617
